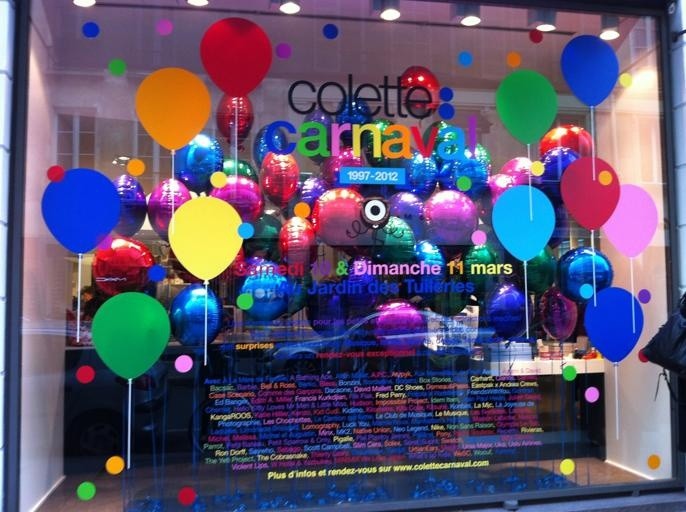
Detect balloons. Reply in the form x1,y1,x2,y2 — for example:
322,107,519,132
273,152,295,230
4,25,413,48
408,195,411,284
73,64,615,386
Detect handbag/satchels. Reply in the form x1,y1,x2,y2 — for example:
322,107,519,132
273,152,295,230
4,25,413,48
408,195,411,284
643,306,685,375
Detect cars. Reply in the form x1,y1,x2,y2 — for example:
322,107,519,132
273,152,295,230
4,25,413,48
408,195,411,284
266,310,497,377
